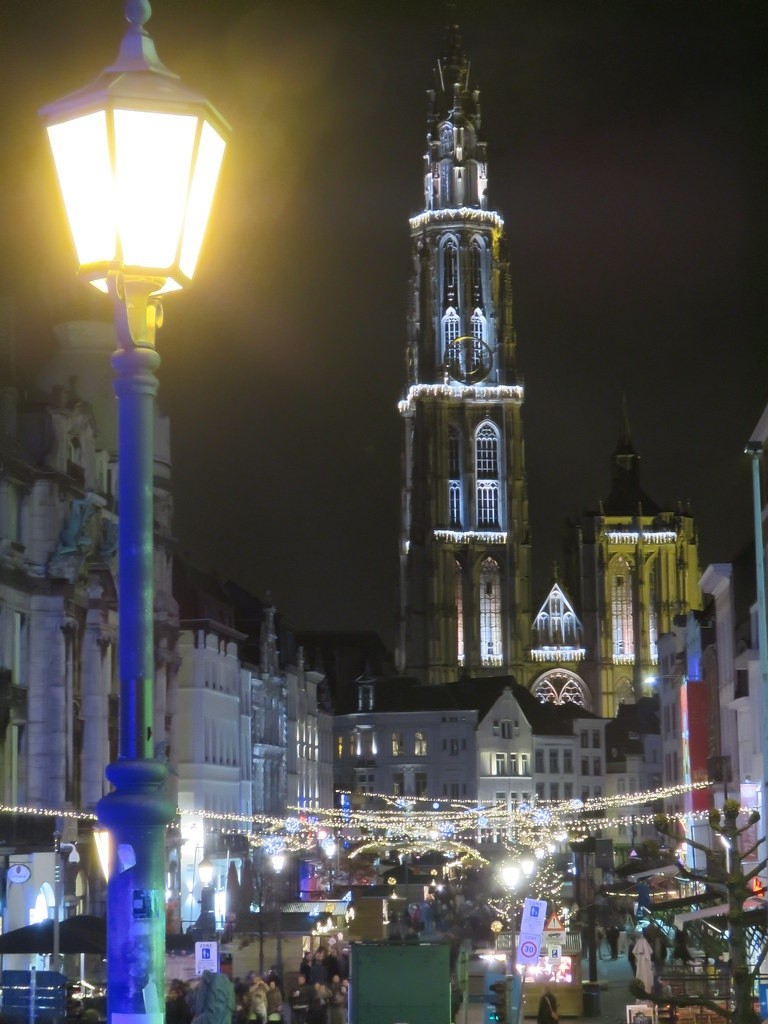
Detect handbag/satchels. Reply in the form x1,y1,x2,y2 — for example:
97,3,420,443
552,1011,558,1020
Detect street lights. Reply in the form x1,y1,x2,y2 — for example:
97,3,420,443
40,0,236,1022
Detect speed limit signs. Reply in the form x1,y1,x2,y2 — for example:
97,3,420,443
522,941,539,958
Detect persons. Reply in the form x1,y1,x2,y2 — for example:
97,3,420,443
582,921,604,960
606,923,619,960
390,891,491,944
627,917,639,961
66,946,349,1024
651,935,667,964
657,976,672,996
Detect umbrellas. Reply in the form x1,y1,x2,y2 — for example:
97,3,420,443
632,937,654,1006
